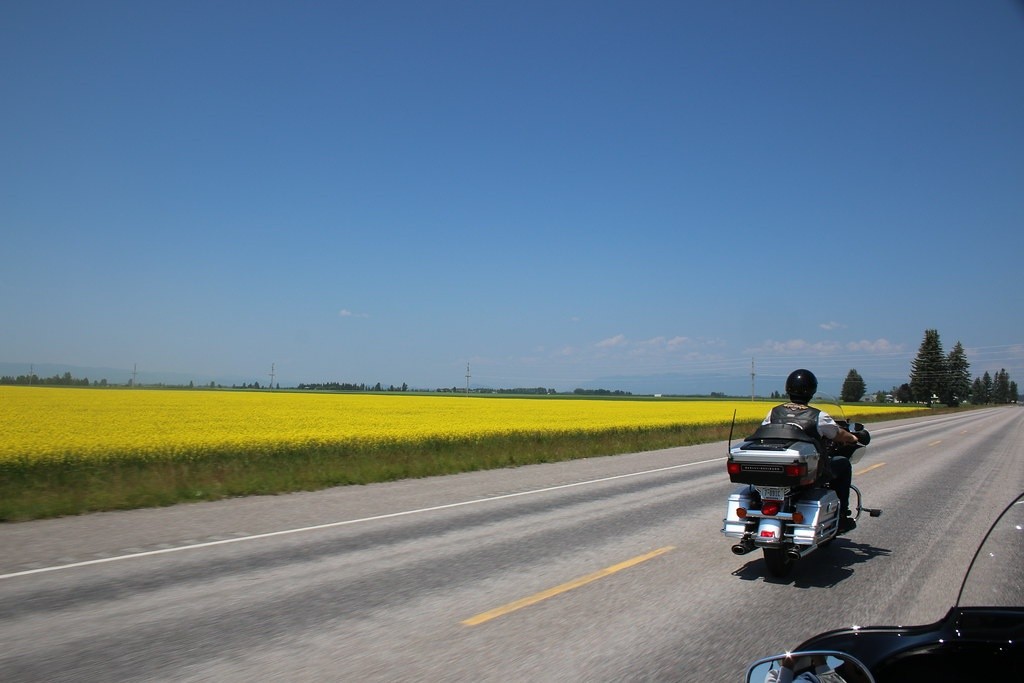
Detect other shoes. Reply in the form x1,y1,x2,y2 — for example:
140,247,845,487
835,517,856,535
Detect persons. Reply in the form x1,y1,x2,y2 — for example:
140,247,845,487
761,369,858,520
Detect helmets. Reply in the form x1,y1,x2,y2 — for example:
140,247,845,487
785,369,818,402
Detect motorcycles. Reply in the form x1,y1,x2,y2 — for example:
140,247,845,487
720,391,882,578
746,493,1024,683
